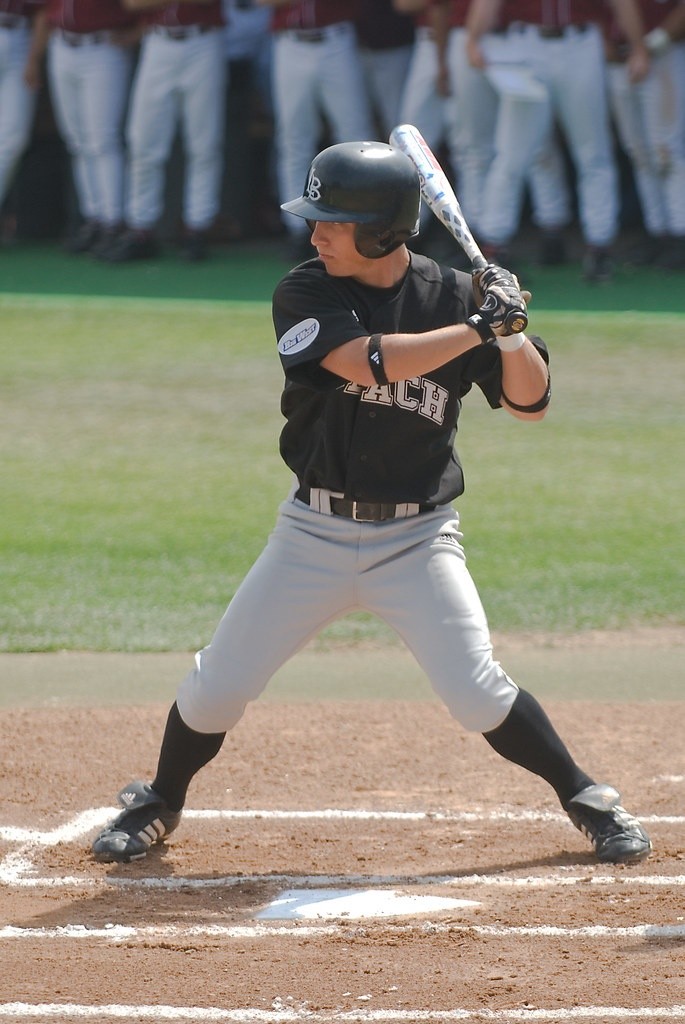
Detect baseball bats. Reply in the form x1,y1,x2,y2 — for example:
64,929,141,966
387,121,531,336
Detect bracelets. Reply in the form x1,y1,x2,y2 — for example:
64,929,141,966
368,332,391,386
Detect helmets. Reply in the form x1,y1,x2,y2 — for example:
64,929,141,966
280,141,421,259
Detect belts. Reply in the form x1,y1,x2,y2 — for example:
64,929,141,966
294,485,437,524
487,25,525,38
298,31,323,42
170,26,209,40
520,24,587,39
0,17,19,28
70,35,100,46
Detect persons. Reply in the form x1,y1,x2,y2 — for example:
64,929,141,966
1,0,685,270
89,140,653,869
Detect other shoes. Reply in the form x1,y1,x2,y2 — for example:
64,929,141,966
281,238,309,262
625,232,661,265
537,236,566,265
67,232,95,252
178,238,207,259
580,244,616,276
656,236,685,266
88,235,109,257
107,231,156,261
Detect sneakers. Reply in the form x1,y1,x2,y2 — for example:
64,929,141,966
92,781,182,863
567,783,653,863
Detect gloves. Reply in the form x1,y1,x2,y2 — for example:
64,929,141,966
465,278,532,346
472,264,526,351
